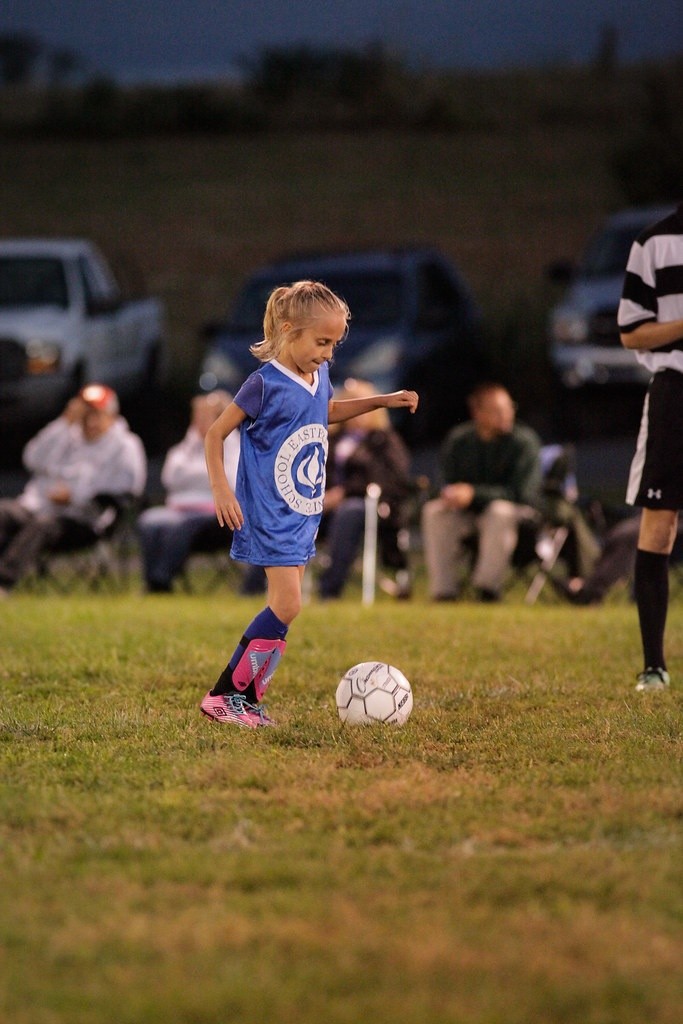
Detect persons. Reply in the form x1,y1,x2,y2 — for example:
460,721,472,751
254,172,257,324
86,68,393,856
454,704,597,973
241,377,411,603
615,207,683,691
418,384,543,603
538,444,643,604
0,384,147,590
137,390,241,595
200,280,419,728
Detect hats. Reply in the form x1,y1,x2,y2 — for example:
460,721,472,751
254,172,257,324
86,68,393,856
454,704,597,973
78,383,119,417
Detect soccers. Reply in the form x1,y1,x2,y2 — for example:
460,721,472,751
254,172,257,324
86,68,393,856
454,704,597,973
335,660,414,727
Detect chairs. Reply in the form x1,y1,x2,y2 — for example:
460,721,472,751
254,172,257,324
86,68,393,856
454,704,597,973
509,446,579,607
14,490,249,595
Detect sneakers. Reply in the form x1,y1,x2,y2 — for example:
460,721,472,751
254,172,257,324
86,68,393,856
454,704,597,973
634,666,670,694
199,689,276,729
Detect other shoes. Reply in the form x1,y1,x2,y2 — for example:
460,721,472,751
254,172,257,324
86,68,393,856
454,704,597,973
435,592,460,604
393,584,410,600
476,589,495,603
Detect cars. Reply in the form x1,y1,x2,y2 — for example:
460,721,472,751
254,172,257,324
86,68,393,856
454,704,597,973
543,205,678,420
192,242,490,459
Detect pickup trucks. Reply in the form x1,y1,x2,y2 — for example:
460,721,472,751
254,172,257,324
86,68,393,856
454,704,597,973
2,238,167,451
